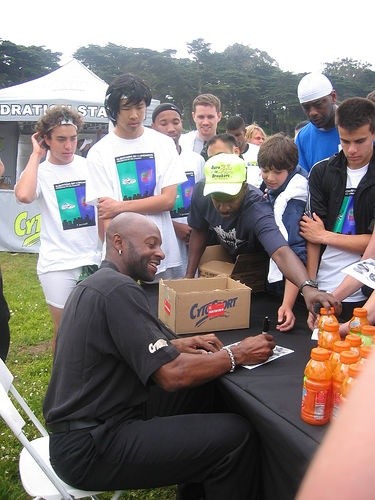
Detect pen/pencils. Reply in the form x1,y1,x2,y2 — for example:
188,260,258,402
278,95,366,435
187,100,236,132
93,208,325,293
261,316,270,336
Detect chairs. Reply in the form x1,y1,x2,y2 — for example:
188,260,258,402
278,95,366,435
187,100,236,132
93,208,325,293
0,357,123,500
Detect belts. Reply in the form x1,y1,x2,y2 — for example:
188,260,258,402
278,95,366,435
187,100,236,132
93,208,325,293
47,417,102,432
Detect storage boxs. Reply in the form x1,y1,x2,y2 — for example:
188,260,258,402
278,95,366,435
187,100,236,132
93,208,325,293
158,278,252,335
198,244,265,293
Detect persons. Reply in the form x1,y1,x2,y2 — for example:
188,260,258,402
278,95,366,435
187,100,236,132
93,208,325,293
293,346,375,500
15,108,103,357
85,73,182,293
152,72,375,338
43,211,277,500
78,129,103,158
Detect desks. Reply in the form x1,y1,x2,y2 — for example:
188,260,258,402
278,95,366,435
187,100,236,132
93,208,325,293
0,189,41,253
143,286,347,500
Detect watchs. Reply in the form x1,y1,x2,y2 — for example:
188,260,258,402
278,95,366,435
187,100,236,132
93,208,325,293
299,279,318,296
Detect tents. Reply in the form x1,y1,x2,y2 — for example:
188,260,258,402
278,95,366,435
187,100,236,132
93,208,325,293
0,57,161,253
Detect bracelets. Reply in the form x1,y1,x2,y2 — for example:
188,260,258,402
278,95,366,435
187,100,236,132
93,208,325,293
222,347,235,372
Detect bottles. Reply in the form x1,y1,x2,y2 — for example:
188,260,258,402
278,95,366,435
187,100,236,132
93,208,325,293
300,307,375,425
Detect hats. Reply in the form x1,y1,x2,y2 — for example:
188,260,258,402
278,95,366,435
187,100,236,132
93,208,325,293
297,73,333,104
202,154,248,195
152,103,182,122
